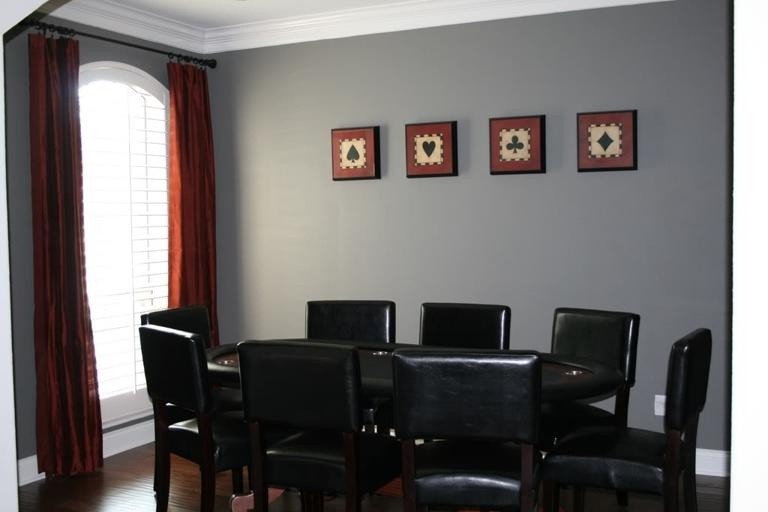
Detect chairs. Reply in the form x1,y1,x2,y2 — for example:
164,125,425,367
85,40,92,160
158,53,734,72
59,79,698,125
390,347,543,512
236,340,381,511
517,307,641,512
399,303,511,508
139,304,230,424
138,324,277,510
540,328,712,512
290,301,396,509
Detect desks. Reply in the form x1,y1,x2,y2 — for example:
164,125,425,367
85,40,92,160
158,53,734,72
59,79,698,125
203,338,626,510
